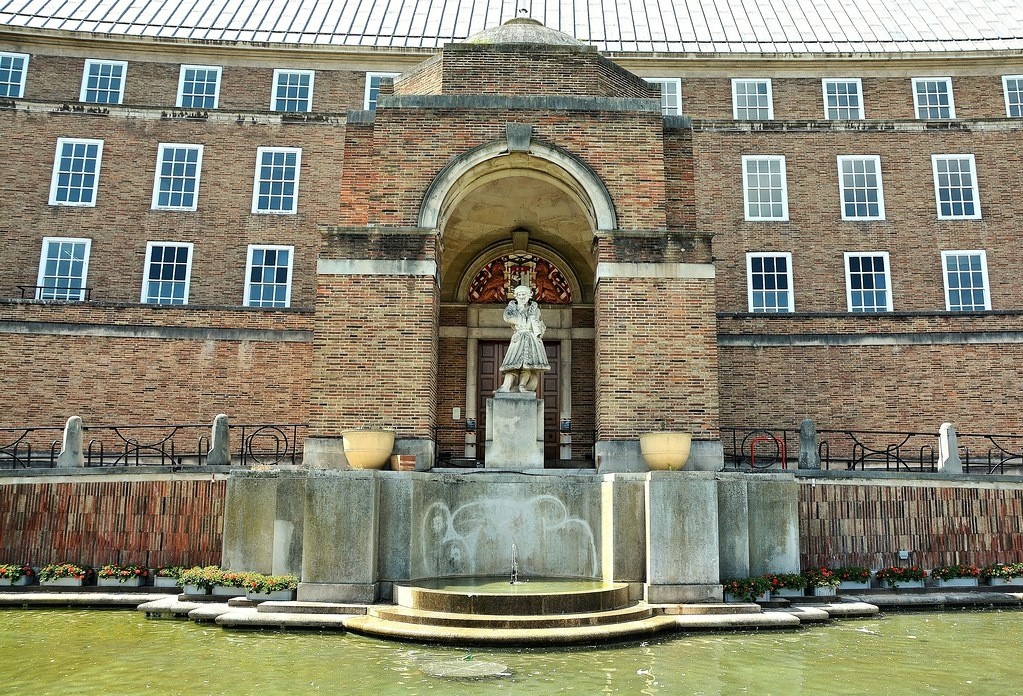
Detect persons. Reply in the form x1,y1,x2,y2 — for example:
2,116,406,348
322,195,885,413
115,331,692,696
492,285,552,396
